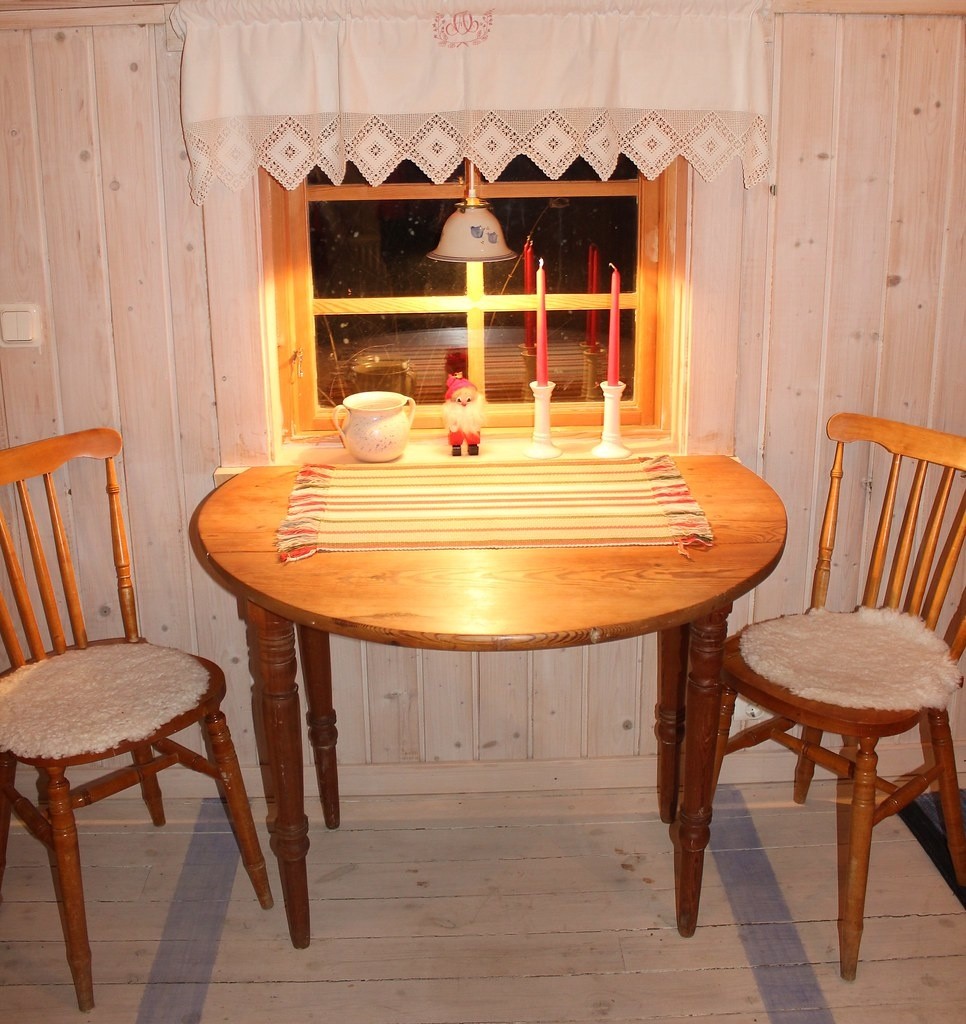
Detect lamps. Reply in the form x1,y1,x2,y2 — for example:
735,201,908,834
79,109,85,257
423,158,517,264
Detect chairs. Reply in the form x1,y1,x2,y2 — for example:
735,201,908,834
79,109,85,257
0,427,277,1015
708,410,966,982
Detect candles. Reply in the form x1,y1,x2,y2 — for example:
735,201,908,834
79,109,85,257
606,262,621,387
524,240,536,349
587,241,599,344
535,258,549,385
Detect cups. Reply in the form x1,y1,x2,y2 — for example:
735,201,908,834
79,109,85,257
334,390,416,460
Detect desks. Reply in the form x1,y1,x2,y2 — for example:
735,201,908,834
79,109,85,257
191,459,788,950
